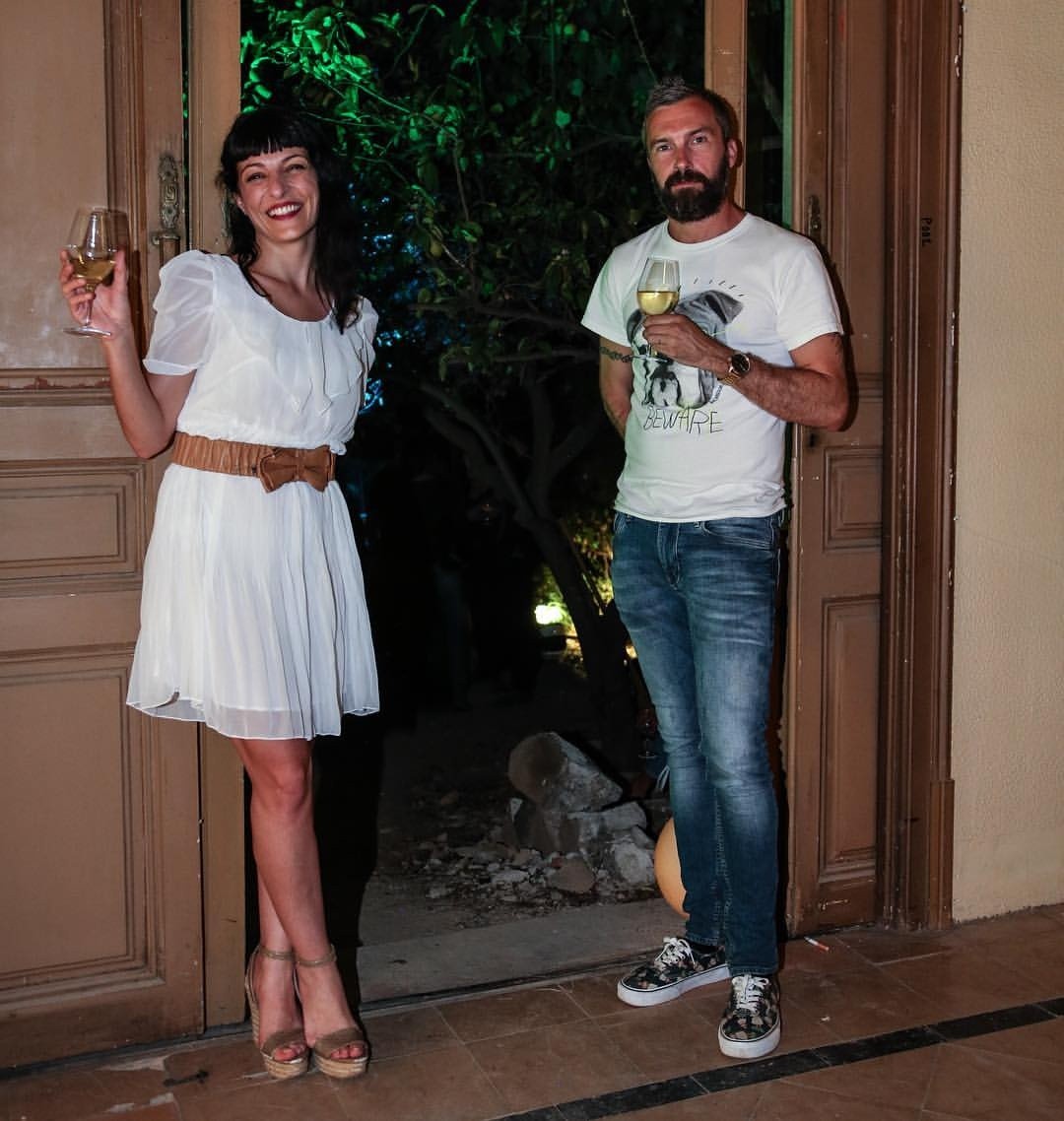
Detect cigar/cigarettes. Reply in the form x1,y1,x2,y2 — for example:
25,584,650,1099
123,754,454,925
803,936,829,951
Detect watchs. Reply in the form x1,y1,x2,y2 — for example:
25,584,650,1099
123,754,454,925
717,348,750,387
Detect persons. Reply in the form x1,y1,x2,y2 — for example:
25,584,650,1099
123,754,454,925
578,76,850,1060
57,103,382,1079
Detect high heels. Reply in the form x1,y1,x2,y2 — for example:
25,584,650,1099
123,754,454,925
243,944,310,1079
293,944,370,1080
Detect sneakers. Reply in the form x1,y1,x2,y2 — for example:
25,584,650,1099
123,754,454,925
718,972,781,1059
617,936,730,1006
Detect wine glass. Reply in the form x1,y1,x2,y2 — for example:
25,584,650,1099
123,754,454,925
63,208,114,340
632,258,680,361
